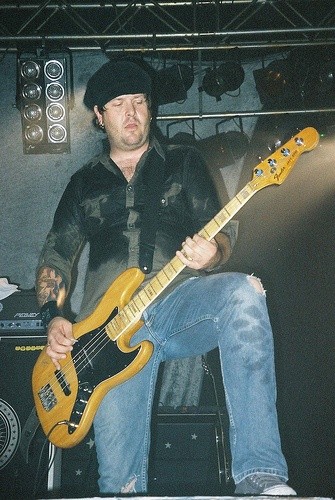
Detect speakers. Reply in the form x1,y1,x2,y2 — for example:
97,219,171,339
151,405,235,496
0,335,63,497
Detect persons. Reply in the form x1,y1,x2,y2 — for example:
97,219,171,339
36,60,296,495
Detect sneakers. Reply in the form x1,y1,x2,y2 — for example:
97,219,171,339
234,473,297,495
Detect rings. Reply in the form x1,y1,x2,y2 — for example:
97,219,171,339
46,344,49,348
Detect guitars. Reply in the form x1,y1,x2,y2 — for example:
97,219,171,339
31,127,320,448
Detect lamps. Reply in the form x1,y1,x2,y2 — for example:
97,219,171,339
146,45,335,170
15,45,74,155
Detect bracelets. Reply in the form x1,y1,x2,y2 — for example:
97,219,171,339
207,250,223,270
40,301,63,328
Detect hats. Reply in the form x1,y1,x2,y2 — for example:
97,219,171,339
83,61,153,109
